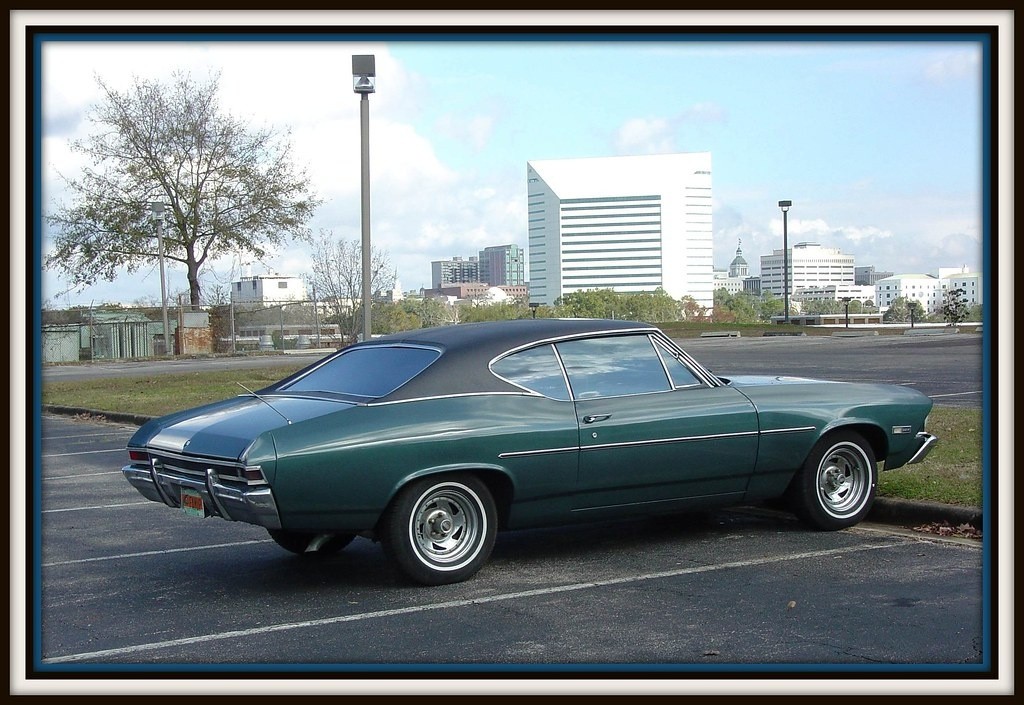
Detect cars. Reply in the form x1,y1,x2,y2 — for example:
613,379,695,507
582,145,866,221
120,316,940,588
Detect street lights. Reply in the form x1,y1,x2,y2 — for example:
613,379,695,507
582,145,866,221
778,200,793,322
351,54,376,348
842,297,851,329
529,303,540,318
151,201,171,354
907,302,917,328
